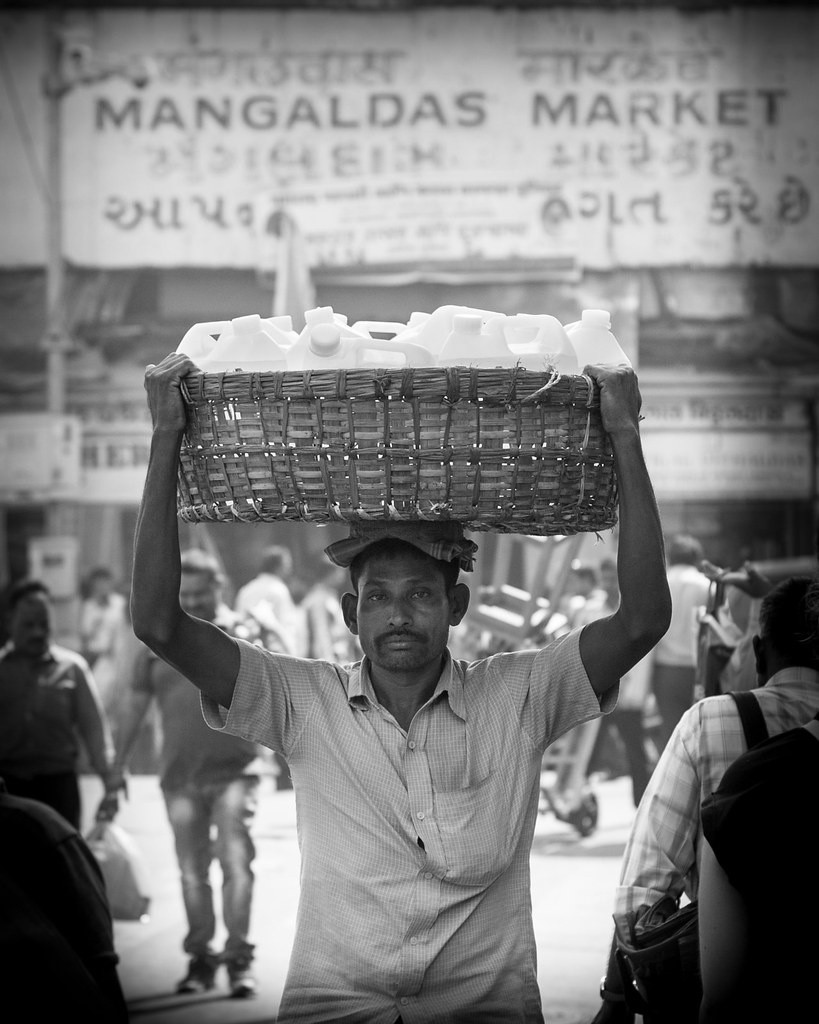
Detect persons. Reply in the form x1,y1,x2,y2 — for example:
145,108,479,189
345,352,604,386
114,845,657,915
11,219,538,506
81,568,128,667
654,537,717,736
113,550,270,998
0,580,124,829
234,544,298,658
0,777,130,1024
612,577,819,1024
569,555,654,806
299,549,357,667
131,351,673,1024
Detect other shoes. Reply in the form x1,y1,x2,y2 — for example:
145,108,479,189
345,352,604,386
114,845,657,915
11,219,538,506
229,958,252,998
176,954,215,993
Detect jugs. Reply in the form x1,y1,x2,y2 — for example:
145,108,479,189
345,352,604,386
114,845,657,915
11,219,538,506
175,307,634,371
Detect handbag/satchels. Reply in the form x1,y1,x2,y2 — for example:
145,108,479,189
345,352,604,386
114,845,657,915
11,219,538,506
617,895,703,1024
83,822,152,923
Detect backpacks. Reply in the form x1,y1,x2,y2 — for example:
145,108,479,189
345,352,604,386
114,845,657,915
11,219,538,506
701,690,819,959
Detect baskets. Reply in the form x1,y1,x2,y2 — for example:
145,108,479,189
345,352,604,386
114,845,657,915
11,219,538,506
176,369,620,534
